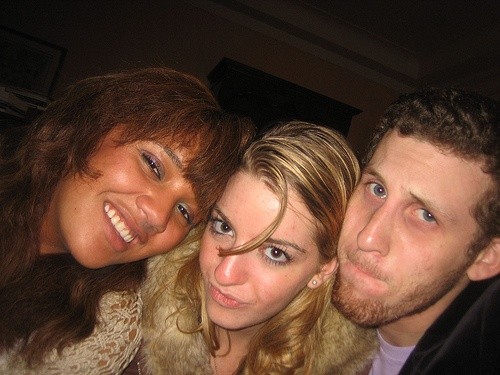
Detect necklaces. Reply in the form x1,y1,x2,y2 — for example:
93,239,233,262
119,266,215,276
212,357,219,374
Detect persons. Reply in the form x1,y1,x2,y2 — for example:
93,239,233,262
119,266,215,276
331,84,500,375
121,122,381,375
0,67,258,375
